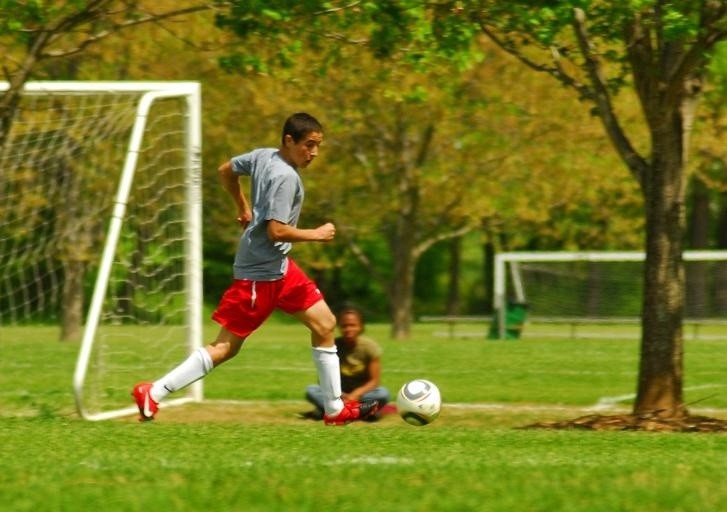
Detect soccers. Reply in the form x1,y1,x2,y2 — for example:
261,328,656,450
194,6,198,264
396,378,441,426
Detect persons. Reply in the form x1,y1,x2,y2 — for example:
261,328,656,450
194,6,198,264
303,307,390,420
130,111,383,426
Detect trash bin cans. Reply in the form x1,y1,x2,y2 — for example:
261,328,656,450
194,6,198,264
489,302,529,339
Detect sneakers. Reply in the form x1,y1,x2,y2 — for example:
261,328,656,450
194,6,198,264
131,383,158,421
324,400,379,426
